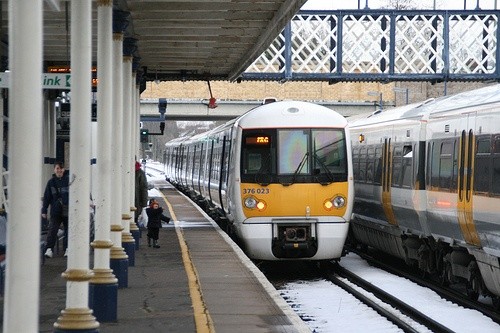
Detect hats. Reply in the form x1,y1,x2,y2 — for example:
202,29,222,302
149,198,159,209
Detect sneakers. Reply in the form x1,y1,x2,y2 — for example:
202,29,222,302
44,248,53,258
64,248,68,256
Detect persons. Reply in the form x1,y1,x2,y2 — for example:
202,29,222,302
135,154,148,223
42,162,69,258
145,198,171,248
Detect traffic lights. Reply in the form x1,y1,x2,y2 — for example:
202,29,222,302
158,98,167,113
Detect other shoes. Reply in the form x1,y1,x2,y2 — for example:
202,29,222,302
148,240,151,247
153,245,160,248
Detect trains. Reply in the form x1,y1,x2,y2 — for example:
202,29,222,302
346,82,499,309
163,100,355,273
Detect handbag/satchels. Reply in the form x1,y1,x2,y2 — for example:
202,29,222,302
137,209,149,229
